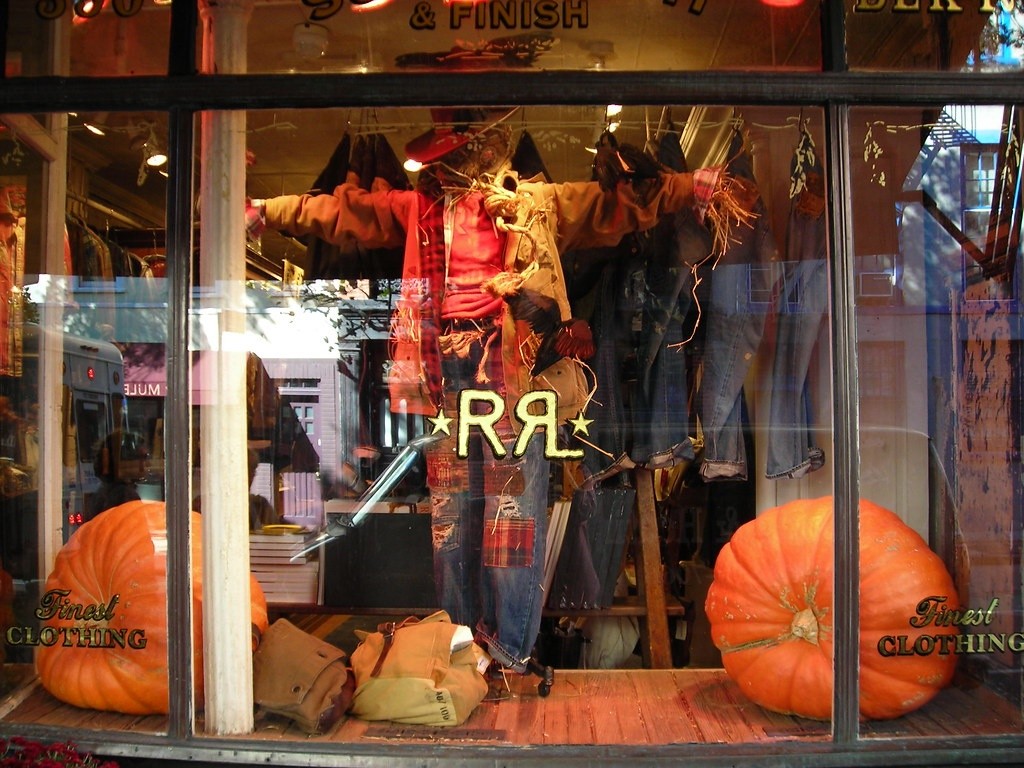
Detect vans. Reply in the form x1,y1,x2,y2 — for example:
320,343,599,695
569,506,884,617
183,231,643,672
288,421,974,671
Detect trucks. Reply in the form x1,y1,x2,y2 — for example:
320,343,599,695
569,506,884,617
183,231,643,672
0,321,133,611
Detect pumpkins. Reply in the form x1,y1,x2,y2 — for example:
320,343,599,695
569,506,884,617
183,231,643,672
573,615,637,669
36,499,268,716
706,495,959,722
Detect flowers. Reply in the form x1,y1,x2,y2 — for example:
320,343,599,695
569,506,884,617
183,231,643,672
0,735,119,768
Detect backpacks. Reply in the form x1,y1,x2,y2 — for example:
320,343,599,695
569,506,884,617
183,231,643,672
348,610,490,726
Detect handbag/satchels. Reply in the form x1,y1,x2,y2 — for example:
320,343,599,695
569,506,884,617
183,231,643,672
251,619,353,736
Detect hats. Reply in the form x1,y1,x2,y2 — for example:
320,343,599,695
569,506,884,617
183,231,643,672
405,107,518,162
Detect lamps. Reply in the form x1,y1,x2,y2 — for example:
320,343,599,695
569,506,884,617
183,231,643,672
145,143,167,166
83,111,108,136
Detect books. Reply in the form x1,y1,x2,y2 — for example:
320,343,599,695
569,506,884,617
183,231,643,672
249,524,320,603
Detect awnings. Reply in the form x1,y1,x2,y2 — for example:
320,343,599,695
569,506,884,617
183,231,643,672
110,341,276,420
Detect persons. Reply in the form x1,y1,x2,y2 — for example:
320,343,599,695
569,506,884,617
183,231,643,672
1,458,38,664
243,105,761,670
79,429,157,657
